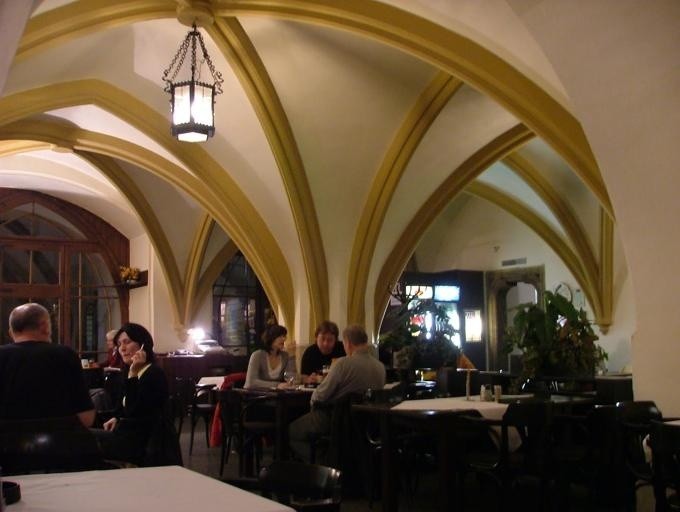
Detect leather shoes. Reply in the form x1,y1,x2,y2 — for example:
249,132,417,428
326,467,342,503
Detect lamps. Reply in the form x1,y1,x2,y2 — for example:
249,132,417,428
161,0,225,145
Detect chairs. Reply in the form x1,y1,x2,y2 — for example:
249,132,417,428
0,366,680,512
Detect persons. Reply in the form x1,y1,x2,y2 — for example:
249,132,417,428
286,324,388,506
243,324,295,479
378,332,478,373
85,322,176,467
0,302,102,473
300,319,347,387
94,329,124,369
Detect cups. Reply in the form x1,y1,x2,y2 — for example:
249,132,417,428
322,365,331,378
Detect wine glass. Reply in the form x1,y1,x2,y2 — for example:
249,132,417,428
284,371,296,390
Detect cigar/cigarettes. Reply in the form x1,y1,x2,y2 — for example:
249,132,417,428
139,342,145,352
